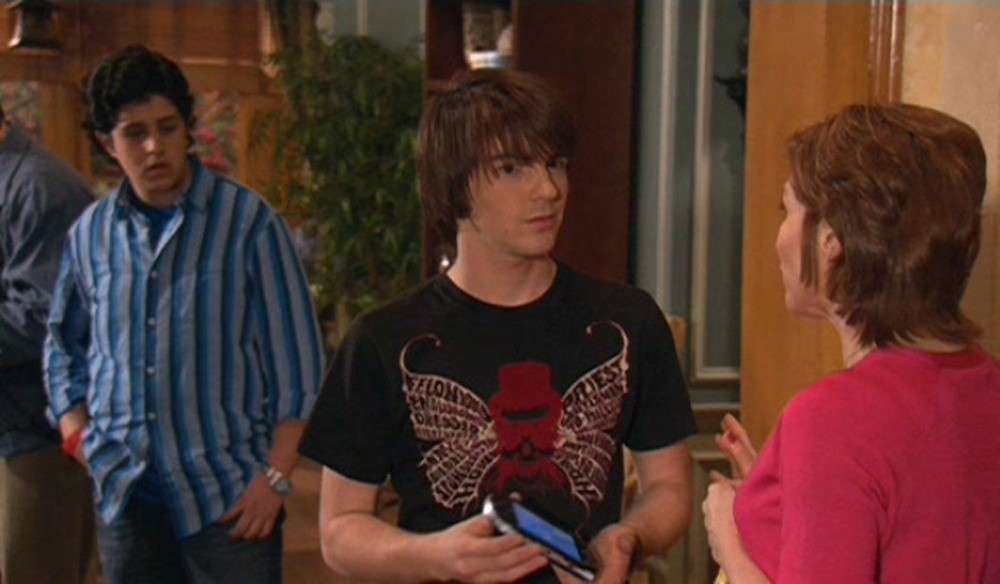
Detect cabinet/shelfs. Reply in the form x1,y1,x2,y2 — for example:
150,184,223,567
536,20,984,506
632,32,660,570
426,0,638,285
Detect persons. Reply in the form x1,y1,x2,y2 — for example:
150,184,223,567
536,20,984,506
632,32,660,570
702,98,1000,584
0,103,99,584
296,67,700,583
42,47,325,584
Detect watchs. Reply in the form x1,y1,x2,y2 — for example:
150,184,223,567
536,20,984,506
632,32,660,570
259,463,292,496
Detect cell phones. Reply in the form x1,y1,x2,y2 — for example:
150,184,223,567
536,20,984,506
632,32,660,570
483,493,600,580
